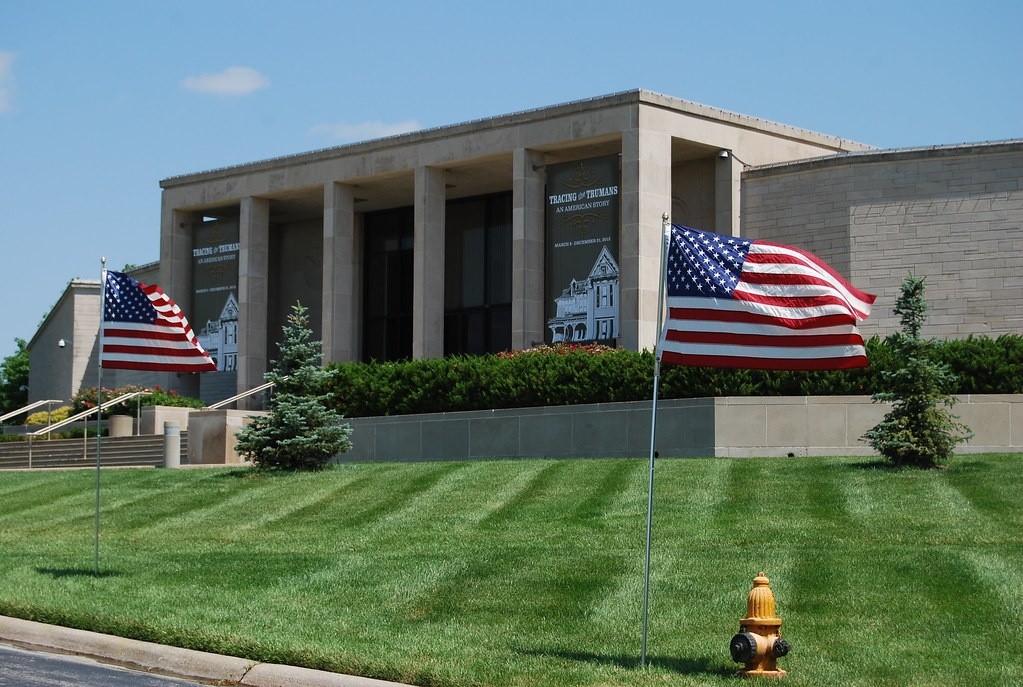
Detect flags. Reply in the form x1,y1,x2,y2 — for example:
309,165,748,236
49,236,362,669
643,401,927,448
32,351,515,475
101,270,219,371
656,222,880,371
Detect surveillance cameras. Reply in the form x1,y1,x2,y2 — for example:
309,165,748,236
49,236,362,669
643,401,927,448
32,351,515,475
717,150,729,159
58,340,66,348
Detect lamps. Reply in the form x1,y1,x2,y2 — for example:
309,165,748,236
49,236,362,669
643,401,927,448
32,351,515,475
532,164,547,173
717,150,729,160
178,222,190,228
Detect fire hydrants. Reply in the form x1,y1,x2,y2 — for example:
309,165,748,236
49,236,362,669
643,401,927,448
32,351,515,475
730,570,792,679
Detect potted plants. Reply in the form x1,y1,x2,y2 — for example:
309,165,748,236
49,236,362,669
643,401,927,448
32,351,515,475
108,391,133,436
